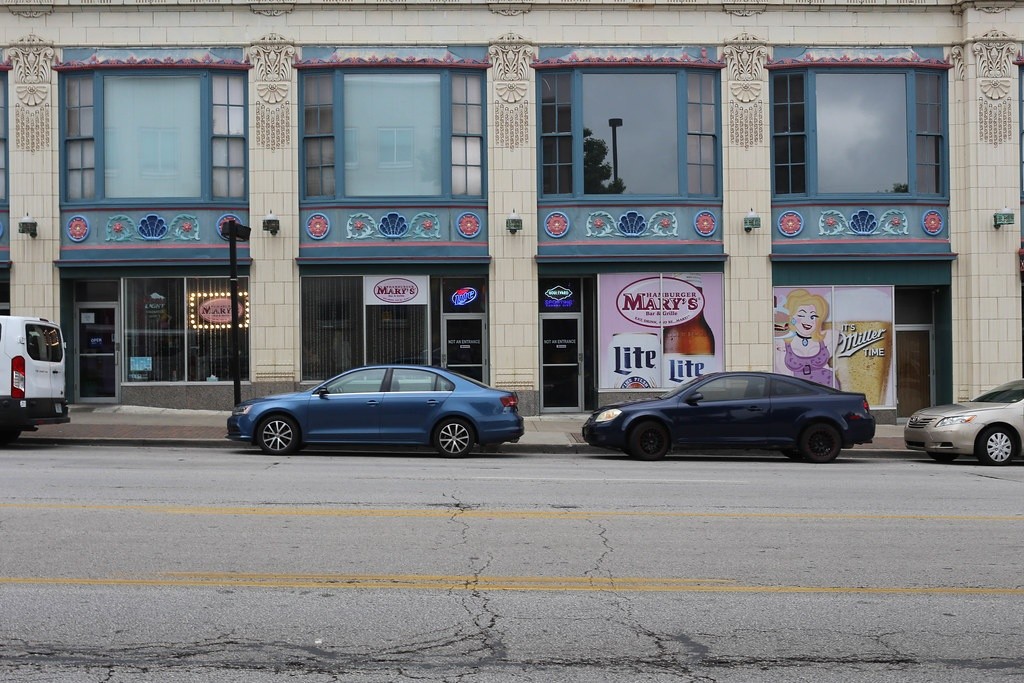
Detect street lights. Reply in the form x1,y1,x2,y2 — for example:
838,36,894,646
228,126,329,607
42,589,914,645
221,219,251,405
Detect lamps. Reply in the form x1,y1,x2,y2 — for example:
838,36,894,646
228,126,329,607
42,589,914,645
18,212,38,239
262,208,280,236
993,205,1014,230
505,208,523,234
743,208,761,233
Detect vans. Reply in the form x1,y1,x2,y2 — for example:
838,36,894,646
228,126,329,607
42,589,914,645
0,315,70,448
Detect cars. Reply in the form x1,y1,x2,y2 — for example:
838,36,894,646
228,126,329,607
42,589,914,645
224,364,525,459
904,379,1024,466
581,370,876,461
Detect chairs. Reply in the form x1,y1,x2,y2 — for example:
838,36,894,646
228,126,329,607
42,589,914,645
392,380,400,392
744,385,761,397
